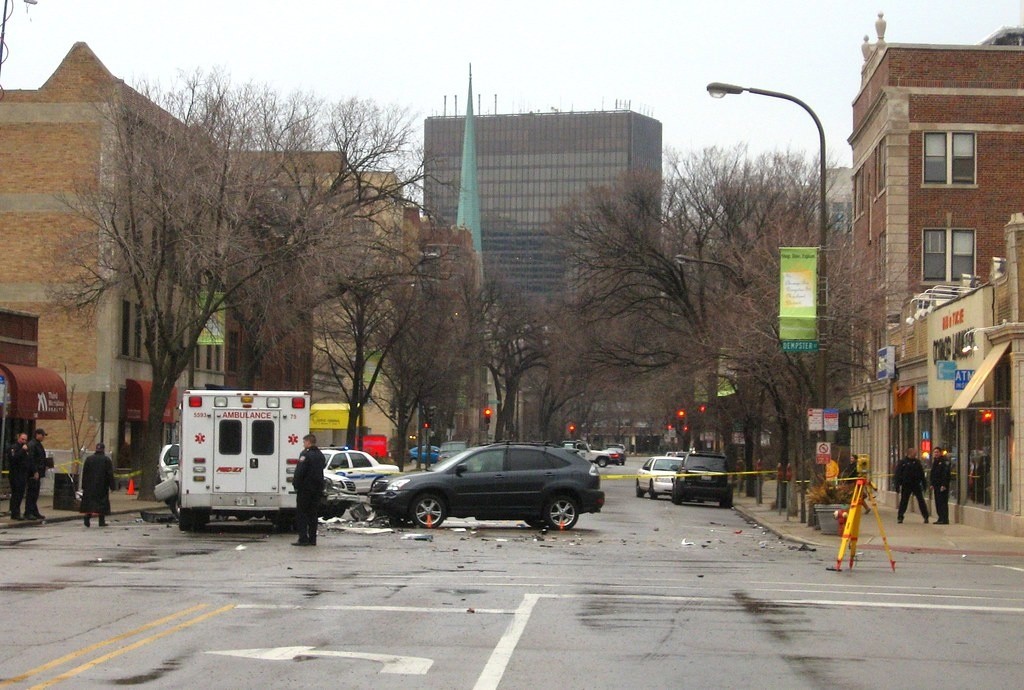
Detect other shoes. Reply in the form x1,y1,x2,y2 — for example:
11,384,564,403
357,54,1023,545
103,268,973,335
898,519,903,523
12,514,24,520
35,513,45,519
924,519,929,523
310,542,316,545
84,516,90,527
24,514,36,520
291,542,308,546
933,520,946,524
100,523,109,526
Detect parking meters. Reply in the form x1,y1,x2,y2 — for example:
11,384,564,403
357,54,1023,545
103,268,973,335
756,458,762,508
735,461,743,496
784,463,793,522
776,463,784,516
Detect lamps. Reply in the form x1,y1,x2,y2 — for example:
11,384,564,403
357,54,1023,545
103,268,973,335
915,293,958,319
961,326,1000,353
919,289,969,315
927,285,975,312
906,298,952,325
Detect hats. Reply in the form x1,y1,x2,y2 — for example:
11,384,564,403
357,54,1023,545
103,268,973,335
36,429,47,436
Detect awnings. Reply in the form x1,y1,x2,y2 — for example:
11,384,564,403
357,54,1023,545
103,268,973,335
126,379,177,423
950,341,1011,410
0,361,67,420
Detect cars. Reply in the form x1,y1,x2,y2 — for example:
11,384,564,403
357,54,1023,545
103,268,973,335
635,456,684,500
561,440,626,468
316,445,400,521
665,451,688,460
408,445,440,464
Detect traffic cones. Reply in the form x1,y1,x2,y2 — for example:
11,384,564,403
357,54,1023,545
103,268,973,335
126,479,136,496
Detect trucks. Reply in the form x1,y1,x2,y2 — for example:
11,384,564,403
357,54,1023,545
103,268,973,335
433,441,467,463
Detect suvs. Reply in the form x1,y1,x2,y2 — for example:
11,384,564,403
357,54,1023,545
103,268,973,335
670,448,737,510
157,443,179,517
366,439,606,531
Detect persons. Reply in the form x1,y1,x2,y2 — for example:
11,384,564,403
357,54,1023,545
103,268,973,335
291,435,325,547
777,461,792,481
895,448,929,523
80,442,116,527
7,432,28,520
929,447,951,525
847,455,858,478
24,428,48,520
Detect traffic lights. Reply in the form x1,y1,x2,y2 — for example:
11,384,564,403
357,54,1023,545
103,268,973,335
404,404,409,419
483,408,492,424
429,407,435,424
389,405,396,419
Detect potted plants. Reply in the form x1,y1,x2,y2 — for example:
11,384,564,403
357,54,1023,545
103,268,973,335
806,475,861,535
54,361,102,511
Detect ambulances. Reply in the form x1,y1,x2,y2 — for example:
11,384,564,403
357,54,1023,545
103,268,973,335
175,387,312,533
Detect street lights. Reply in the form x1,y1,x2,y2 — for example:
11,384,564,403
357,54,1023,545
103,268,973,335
357,250,441,451
0,0,41,75
706,79,831,531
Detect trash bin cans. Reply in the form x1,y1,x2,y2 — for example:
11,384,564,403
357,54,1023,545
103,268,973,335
54,473,78,509
